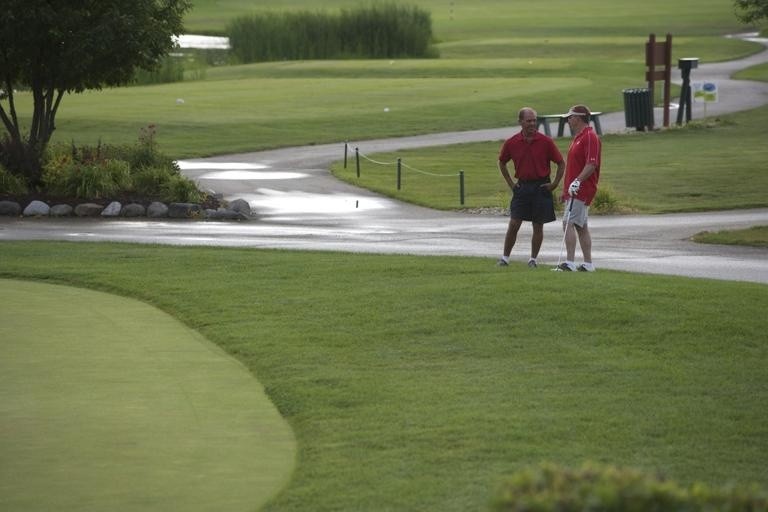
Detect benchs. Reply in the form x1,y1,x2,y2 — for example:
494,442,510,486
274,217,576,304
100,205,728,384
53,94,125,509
534,111,604,139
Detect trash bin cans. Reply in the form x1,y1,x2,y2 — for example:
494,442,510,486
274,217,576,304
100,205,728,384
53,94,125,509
622,88,654,132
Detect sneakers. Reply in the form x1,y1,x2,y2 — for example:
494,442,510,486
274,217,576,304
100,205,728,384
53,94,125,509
577,263,595,272
497,259,508,266
551,262,576,272
528,260,536,268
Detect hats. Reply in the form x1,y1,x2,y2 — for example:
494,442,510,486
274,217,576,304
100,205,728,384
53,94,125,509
560,106,590,118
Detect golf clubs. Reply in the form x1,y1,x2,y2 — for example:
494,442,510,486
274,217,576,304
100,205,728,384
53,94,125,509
550,194,574,272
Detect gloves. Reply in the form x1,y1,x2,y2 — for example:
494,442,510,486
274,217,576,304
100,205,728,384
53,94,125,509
568,178,580,196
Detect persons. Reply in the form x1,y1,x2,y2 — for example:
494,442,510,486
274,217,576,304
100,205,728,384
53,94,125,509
497,108,566,269
557,106,601,273
